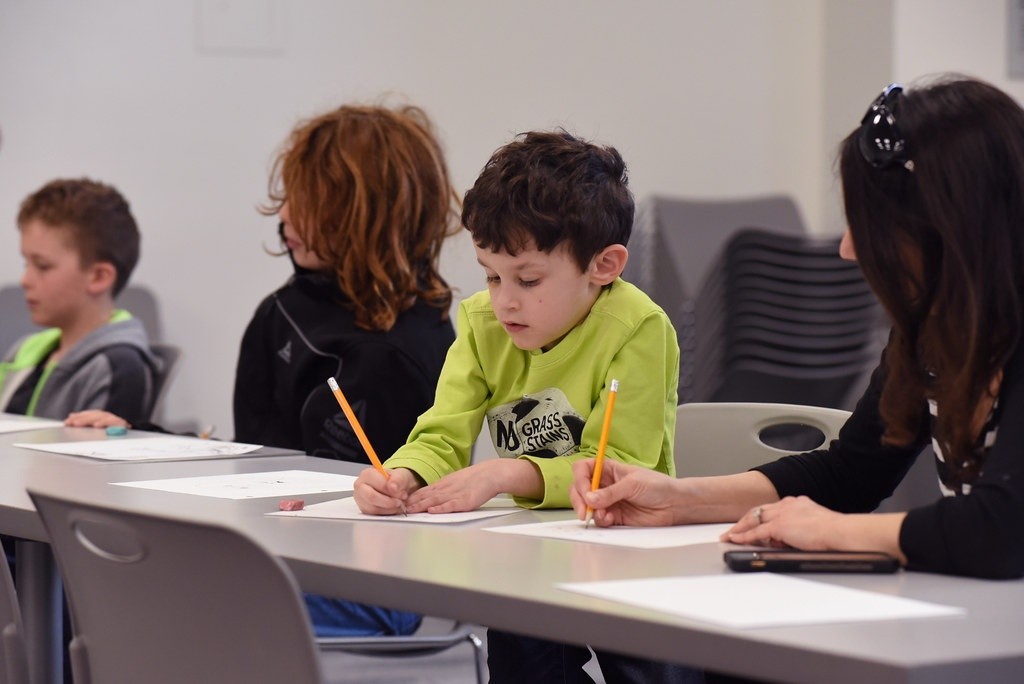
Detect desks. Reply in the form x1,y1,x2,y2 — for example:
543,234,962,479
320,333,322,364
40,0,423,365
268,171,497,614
0,399,1023,684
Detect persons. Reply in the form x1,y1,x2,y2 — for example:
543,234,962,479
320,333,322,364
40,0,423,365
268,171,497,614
0,177,164,422
353,128,680,683
571,75,1023,581
67,105,462,635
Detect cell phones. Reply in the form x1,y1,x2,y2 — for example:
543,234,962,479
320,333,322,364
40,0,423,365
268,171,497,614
724,550,901,575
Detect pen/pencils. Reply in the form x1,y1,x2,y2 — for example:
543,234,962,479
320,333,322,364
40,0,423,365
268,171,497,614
586,379,619,528
327,376,408,517
200,425,214,440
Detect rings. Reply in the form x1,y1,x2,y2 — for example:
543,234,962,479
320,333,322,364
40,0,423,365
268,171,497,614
755,507,763,525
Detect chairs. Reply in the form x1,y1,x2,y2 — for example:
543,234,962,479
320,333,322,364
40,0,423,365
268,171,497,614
142,342,184,428
24,483,334,682
622,191,892,477
317,297,483,684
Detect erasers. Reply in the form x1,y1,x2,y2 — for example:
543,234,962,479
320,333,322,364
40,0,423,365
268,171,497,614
279,499,305,511
105,426,127,436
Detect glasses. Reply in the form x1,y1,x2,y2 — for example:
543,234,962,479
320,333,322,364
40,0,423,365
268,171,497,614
861,84,917,179
